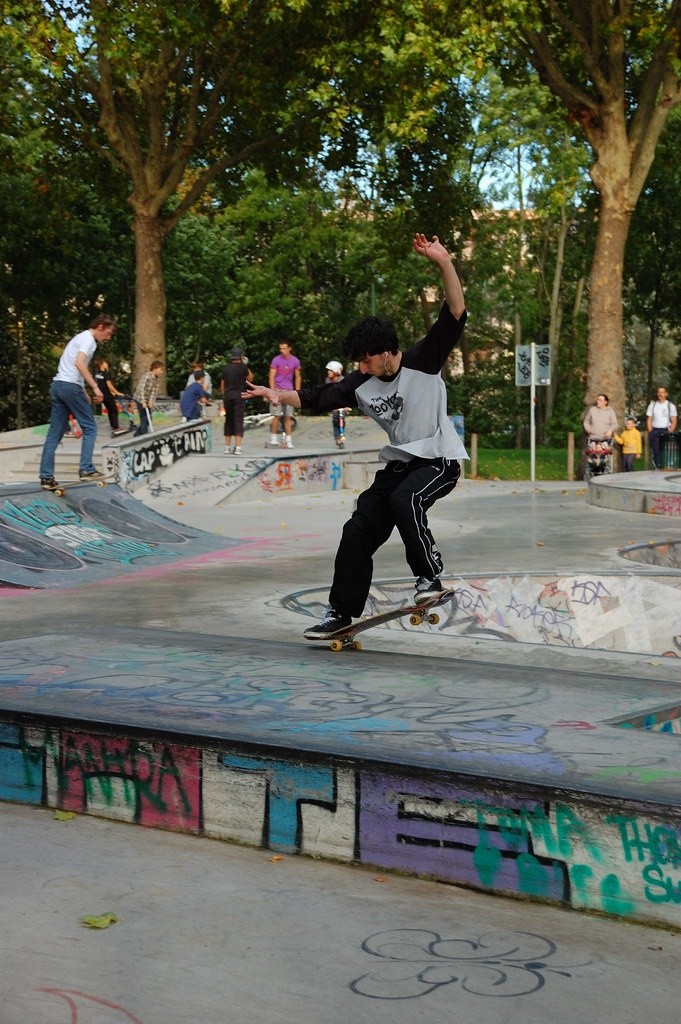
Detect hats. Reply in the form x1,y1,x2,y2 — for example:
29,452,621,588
230,348,244,360
195,370,208,378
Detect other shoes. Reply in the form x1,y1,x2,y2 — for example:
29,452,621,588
270,437,279,445
226,447,232,453
234,447,243,455
285,439,293,449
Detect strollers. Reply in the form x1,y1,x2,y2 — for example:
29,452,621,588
583,433,614,481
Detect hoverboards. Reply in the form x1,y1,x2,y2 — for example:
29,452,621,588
112,394,137,438
332,408,352,450
63,414,83,438
198,402,210,417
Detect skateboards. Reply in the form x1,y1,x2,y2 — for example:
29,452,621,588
48,472,117,496
319,589,457,652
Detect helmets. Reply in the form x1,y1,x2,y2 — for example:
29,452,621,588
325,360,343,376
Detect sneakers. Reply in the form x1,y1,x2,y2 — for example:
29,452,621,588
41,478,58,490
413,575,441,604
304,607,352,639
79,469,104,481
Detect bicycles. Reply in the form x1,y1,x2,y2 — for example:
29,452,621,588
242,410,296,432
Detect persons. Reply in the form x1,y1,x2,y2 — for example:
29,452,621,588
220,347,254,454
39,314,116,489
240,231,468,639
325,360,351,443
268,341,302,449
583,386,677,478
94,357,213,436
